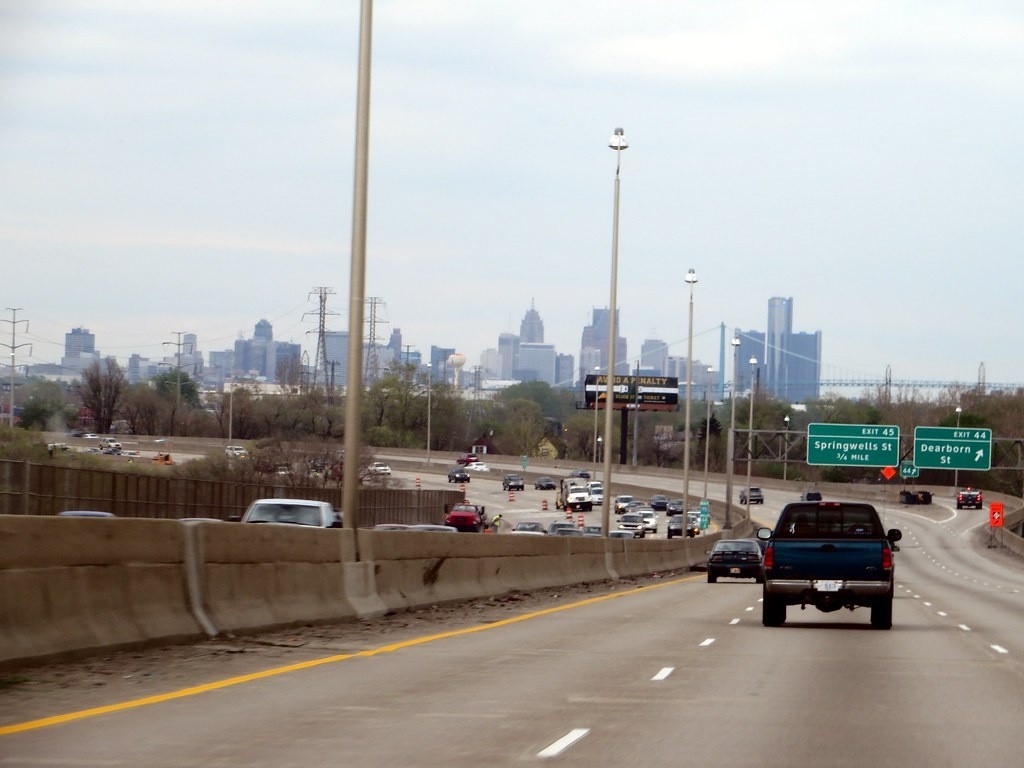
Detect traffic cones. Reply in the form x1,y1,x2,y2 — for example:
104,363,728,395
416,477,421,487
509,492,515,501
542,500,547,510
566,507,573,521
460,484,465,491
577,514,584,527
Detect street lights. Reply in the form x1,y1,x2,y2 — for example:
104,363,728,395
228,383,233,439
954,406,962,487
681,267,699,542
425,363,433,464
600,126,630,541
746,357,758,517
724,338,741,527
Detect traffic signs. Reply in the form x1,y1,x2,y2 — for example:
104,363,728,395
913,426,991,471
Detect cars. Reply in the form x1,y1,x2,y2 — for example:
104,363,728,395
799,492,822,502
739,487,763,504
957,487,982,510
705,538,765,584
56,428,713,540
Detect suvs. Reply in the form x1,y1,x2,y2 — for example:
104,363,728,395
756,501,903,629
